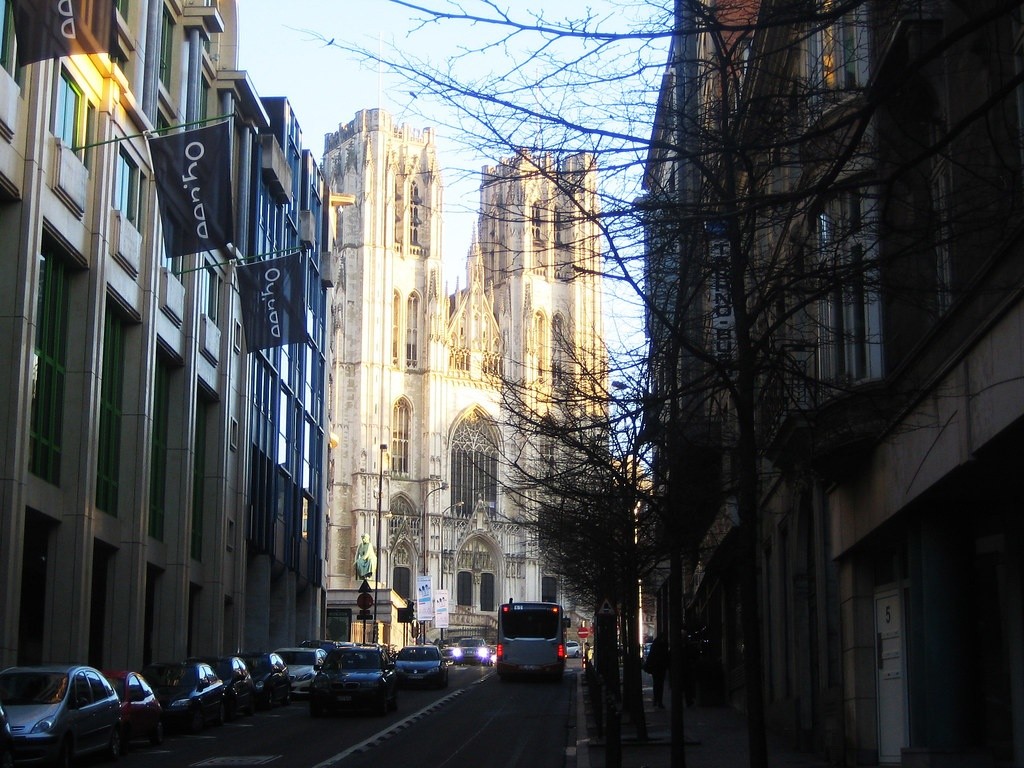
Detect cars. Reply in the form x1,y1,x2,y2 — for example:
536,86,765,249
139,659,227,735
189,655,257,721
566,639,582,658
0,664,125,768
99,669,166,754
272,646,330,695
642,643,653,658
298,639,363,663
440,637,496,667
233,651,292,710
391,644,452,690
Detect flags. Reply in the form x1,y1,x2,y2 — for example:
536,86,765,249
145,123,236,260
235,253,313,351
8,0,115,65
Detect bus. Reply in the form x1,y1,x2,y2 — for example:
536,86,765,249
496,597,571,684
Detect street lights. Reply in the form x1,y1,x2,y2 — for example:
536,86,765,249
423,484,449,644
440,502,466,641
371,444,389,645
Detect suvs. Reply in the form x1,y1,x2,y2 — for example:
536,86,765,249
308,646,400,716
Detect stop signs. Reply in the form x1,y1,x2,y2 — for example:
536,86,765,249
577,627,589,638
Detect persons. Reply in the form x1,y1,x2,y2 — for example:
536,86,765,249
586,644,594,665
353,534,377,582
644,626,698,708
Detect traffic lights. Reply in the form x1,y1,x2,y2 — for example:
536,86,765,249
406,601,415,622
